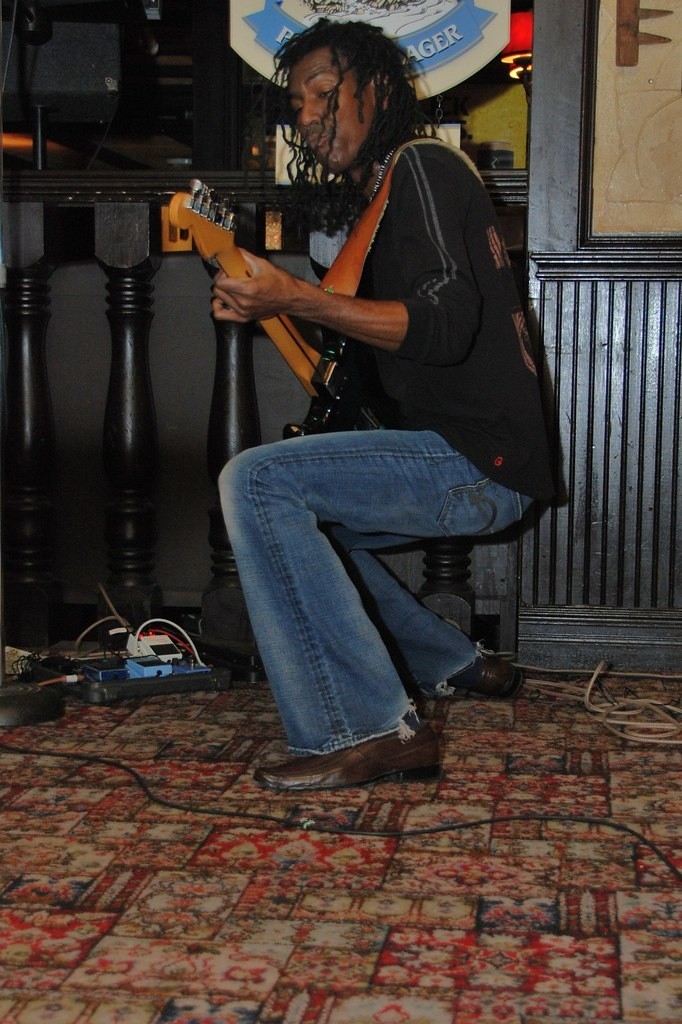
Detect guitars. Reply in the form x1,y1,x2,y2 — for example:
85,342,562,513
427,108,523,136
166,176,396,440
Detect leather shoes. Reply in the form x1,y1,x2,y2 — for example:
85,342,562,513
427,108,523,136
253,720,442,790
449,655,524,700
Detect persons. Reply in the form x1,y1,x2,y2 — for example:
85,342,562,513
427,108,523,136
211,17,557,794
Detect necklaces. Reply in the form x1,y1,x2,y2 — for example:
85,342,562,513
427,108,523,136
369,148,397,206
